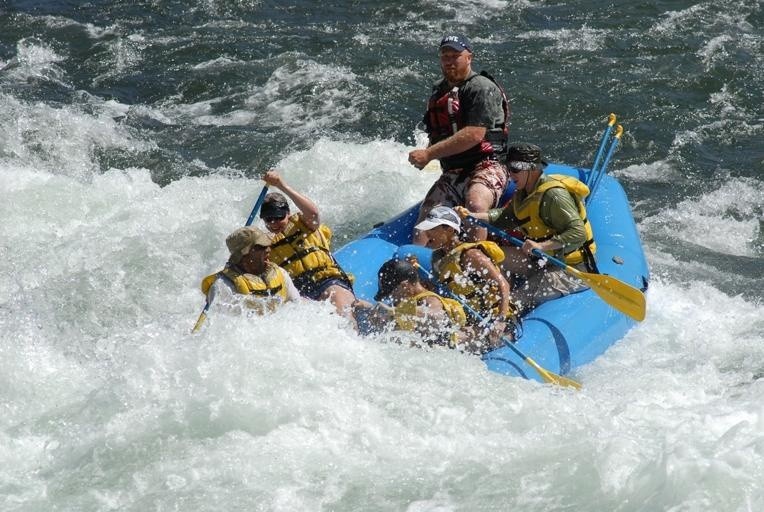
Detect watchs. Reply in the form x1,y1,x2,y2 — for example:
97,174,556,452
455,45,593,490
495,314,507,321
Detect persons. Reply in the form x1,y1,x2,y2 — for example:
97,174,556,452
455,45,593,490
409,32,510,244
202,227,301,315
453,142,597,316
353,258,467,350
414,207,524,354
259,172,356,319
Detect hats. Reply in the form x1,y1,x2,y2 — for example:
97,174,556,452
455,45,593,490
442,32,474,54
259,192,288,219
373,259,416,301
415,206,462,233
499,142,548,171
225,226,278,263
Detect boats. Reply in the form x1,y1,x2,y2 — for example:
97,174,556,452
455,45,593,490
331,163,650,390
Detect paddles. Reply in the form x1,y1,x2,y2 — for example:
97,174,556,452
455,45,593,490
413,261,583,393
483,219,645,323
190,180,270,335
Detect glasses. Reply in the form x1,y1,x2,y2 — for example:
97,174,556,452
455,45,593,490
508,165,522,174
266,215,285,222
